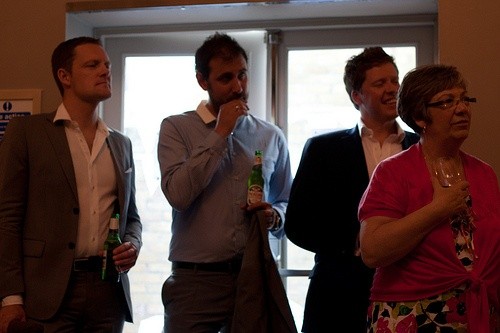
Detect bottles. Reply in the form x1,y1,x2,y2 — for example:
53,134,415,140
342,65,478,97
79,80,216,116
102,213,123,283
246,150,265,209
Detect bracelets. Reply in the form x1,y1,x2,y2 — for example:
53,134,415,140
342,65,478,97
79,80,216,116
271,213,281,231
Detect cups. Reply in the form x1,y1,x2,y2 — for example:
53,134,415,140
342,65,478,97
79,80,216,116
436,154,465,187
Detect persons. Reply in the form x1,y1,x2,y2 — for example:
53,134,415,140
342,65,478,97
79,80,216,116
158,32,297,332
0,36,142,333
358,65,500,332
285,55,421,332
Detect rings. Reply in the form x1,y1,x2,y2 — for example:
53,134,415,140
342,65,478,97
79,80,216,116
235,105,239,111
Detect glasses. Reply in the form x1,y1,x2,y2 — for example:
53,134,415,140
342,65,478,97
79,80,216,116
423,93,477,109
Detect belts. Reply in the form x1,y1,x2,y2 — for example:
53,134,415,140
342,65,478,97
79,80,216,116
174,260,242,271
73,258,105,274
